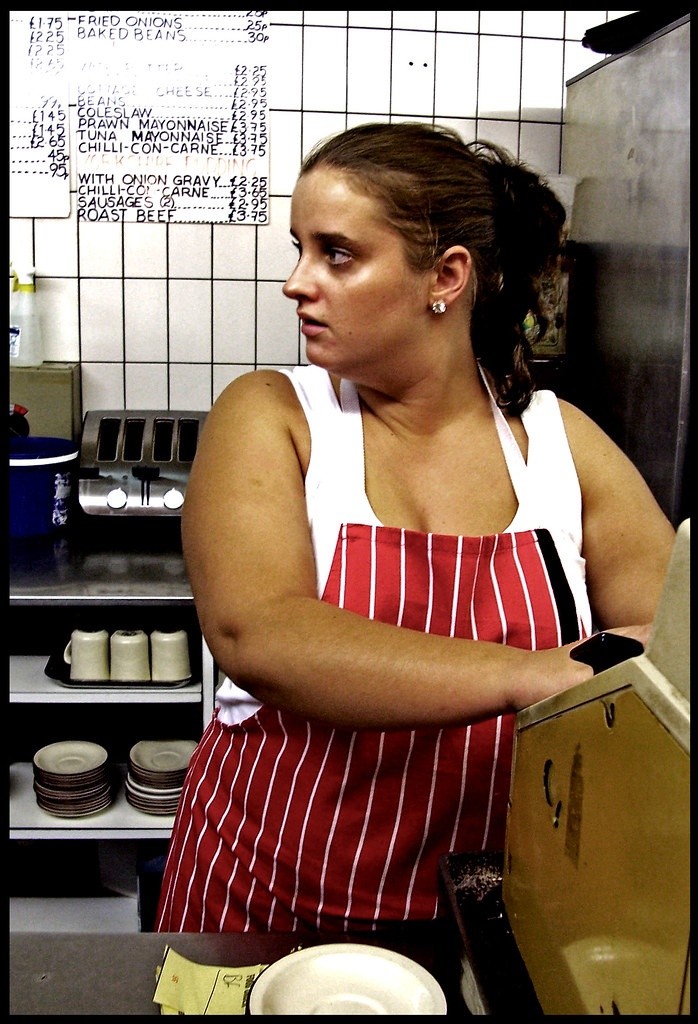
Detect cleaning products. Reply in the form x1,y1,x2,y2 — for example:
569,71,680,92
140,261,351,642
8,263,40,369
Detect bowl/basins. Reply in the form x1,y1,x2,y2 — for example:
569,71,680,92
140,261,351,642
249,943,447,1015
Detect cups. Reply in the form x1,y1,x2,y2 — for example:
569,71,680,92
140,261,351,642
63,628,109,681
111,630,152,682
149,628,192,683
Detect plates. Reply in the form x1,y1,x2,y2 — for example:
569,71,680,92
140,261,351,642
124,740,199,815
32,740,114,817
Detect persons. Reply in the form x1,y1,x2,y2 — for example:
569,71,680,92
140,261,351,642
154,122,676,932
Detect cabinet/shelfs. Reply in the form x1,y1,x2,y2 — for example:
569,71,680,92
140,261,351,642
8,549,217,838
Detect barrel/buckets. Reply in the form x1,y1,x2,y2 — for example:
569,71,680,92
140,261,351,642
8,435,82,537
